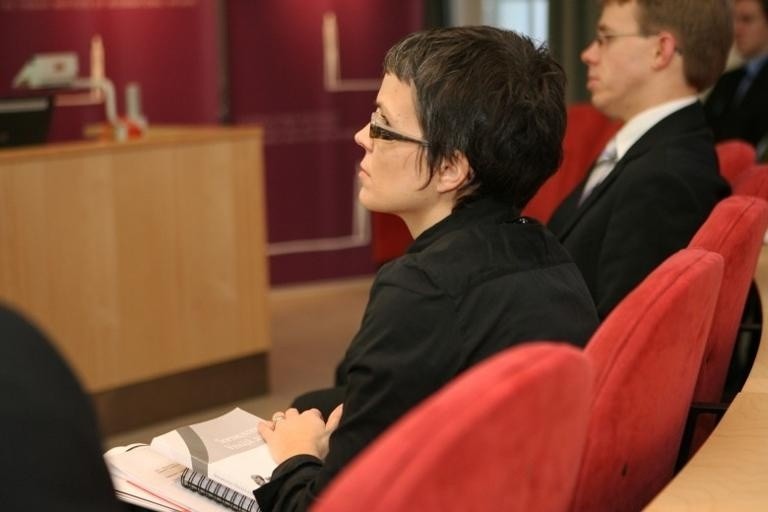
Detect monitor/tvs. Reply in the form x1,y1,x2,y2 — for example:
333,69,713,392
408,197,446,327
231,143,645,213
0,93,55,148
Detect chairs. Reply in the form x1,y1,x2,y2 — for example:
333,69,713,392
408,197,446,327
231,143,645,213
302,101,767,511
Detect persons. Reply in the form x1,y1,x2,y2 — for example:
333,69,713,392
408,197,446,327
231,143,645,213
549,1,734,314
703,1,768,148
0,302,114,512
257,25,599,512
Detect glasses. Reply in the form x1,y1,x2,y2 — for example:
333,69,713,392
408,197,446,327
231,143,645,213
595,30,676,39
369,110,431,147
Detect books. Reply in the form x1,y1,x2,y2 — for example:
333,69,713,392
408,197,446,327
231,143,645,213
103,407,277,511
